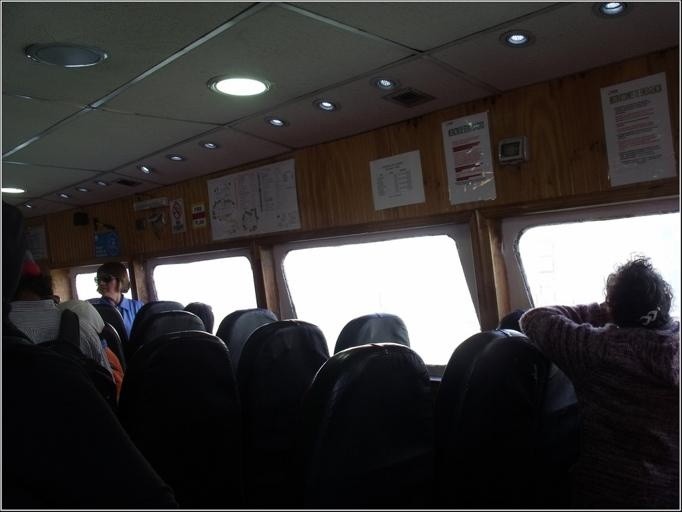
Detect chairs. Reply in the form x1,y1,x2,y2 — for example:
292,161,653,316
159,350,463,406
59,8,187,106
2,301,583,509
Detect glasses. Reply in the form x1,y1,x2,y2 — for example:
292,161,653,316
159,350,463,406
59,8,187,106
94,275,112,284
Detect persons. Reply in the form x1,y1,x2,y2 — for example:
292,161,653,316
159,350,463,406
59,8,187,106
495,309,526,333
2,202,178,508
519,259,679,509
12,261,214,400
